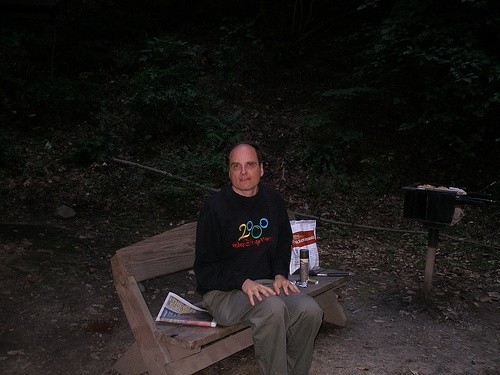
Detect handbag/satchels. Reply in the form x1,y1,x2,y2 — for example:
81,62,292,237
287,219,321,275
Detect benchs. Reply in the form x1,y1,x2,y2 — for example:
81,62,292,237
111,222,348,375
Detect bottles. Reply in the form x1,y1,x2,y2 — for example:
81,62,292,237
300,249,309,283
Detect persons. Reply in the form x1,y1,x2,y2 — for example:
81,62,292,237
195,143,322,375
